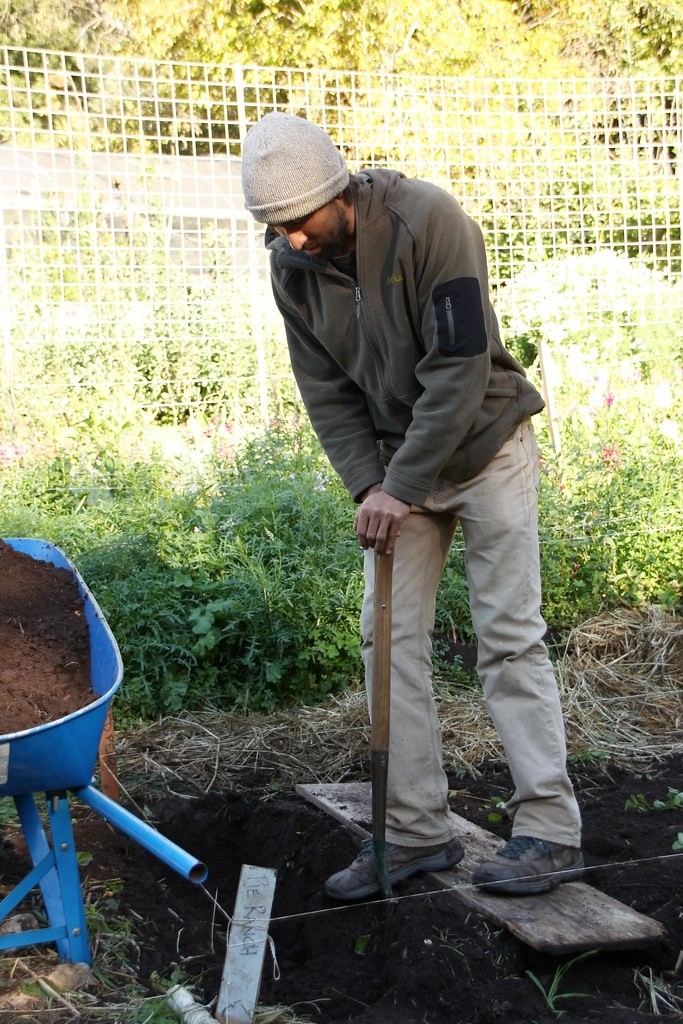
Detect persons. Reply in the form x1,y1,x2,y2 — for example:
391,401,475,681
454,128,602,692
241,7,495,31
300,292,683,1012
241,113,583,893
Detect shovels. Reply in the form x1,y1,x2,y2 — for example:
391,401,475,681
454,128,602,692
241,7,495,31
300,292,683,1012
368,525,399,959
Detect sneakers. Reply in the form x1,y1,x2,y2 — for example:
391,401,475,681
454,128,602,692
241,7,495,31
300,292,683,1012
472,837,585,892
326,834,464,900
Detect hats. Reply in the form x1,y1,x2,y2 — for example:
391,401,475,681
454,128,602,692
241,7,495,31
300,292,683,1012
241,112,350,225
